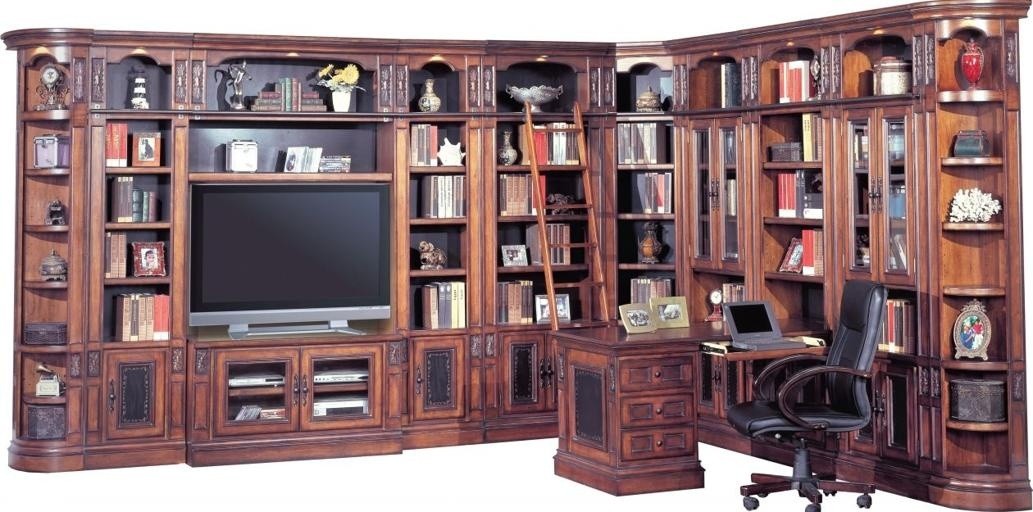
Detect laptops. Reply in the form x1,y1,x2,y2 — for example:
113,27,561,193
721,301,806,351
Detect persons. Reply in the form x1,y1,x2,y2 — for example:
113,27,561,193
227,60,251,109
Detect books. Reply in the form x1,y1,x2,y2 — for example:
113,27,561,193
407,59,915,360
253,76,352,173
104,123,171,342
236,404,286,421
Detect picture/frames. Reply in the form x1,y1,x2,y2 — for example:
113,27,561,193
952,297,992,361
535,294,571,325
130,240,165,276
649,295,690,329
501,244,529,267
131,131,160,167
778,237,803,274
618,303,658,335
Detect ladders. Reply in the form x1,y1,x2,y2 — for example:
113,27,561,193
524,100,611,332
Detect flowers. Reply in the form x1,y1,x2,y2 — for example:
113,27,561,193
949,187,1003,223
317,61,367,94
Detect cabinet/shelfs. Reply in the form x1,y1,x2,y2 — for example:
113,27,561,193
408,333,557,447
186,32,393,179
1,27,186,471
397,40,485,334
838,1,1030,281
186,337,409,469
484,40,615,333
674,21,803,272
615,41,675,327
842,280,1030,510
698,344,746,453
755,19,837,273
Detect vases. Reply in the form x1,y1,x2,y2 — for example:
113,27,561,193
332,91,351,112
498,132,518,165
417,78,442,112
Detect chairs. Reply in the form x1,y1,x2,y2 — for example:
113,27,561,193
726,279,889,511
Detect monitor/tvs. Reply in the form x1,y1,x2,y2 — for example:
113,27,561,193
189,183,389,340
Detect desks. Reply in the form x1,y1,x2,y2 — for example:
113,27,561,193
550,316,831,495
